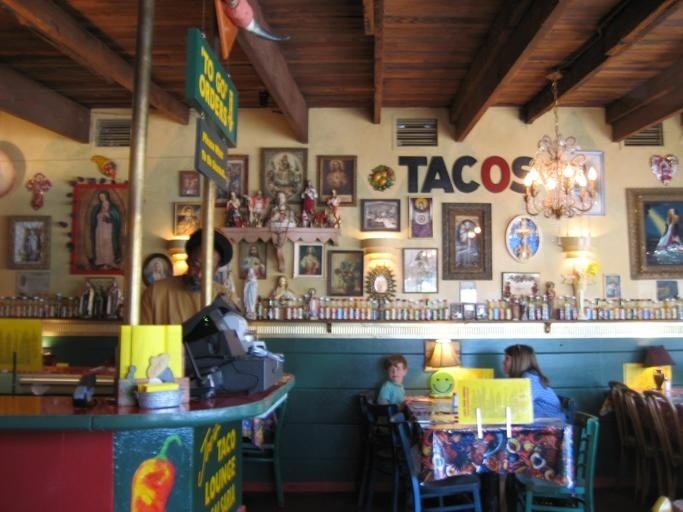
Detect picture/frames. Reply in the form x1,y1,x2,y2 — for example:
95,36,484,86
422,339,460,373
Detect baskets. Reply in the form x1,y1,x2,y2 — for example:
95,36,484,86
135,387,184,408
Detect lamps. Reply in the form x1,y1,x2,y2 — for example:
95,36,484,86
427,337,461,398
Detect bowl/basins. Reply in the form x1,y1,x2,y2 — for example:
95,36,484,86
130,387,189,410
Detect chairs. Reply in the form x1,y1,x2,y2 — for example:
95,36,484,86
514,379,683,512
353,388,482,512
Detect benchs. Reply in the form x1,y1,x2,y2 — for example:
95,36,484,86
240,396,289,510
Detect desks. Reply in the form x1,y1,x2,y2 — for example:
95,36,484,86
403,394,574,512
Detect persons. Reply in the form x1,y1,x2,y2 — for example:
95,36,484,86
267,276,298,302
146,261,168,285
89,190,120,270
376,351,432,484
139,226,236,327
242,266,259,320
25,229,38,260
221,153,351,235
242,245,264,278
300,247,321,275
500,343,568,422
102,280,122,320
77,279,96,320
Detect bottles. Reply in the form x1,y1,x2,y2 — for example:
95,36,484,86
0,291,682,321
